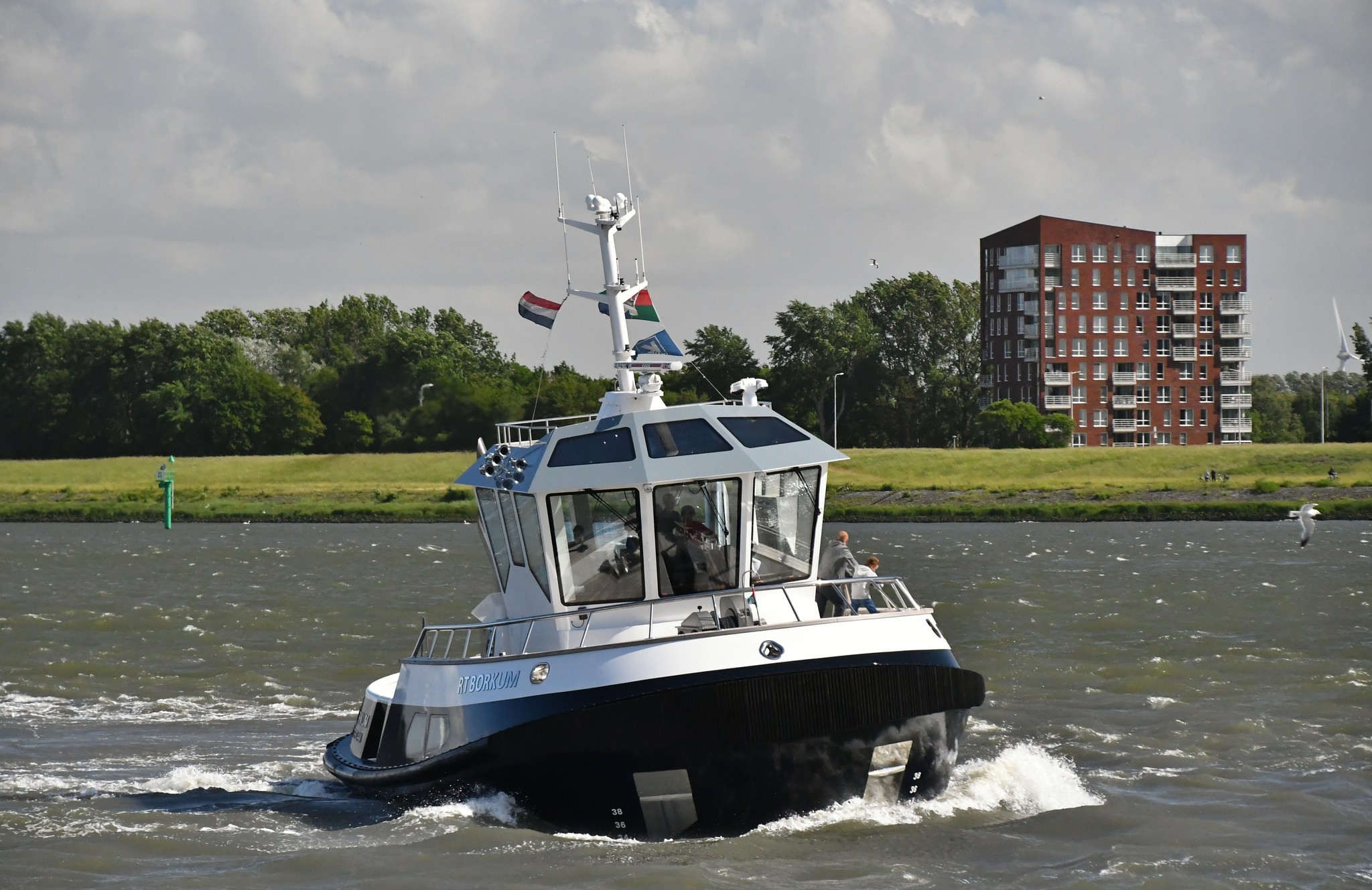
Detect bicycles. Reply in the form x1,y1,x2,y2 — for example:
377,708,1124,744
1215,474,1227,483
1197,473,1211,482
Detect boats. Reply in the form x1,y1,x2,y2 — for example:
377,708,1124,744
320,186,988,837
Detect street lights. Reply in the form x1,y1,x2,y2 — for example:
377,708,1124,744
1320,366,1329,443
834,373,845,449
420,383,434,406
952,436,957,448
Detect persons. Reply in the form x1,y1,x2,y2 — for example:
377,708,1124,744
1211,469,1216,482
630,329,684,360
815,531,858,618
850,557,884,615
598,287,660,323
1204,470,1210,482
1329,466,1337,480
568,525,587,549
624,493,717,540
518,291,561,330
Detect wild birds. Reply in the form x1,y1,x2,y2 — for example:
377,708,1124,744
1037,96,1046,100
869,258,879,269
1287,501,1323,551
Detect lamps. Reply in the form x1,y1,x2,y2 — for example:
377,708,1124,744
493,458,528,490
697,479,707,487
479,445,511,478
